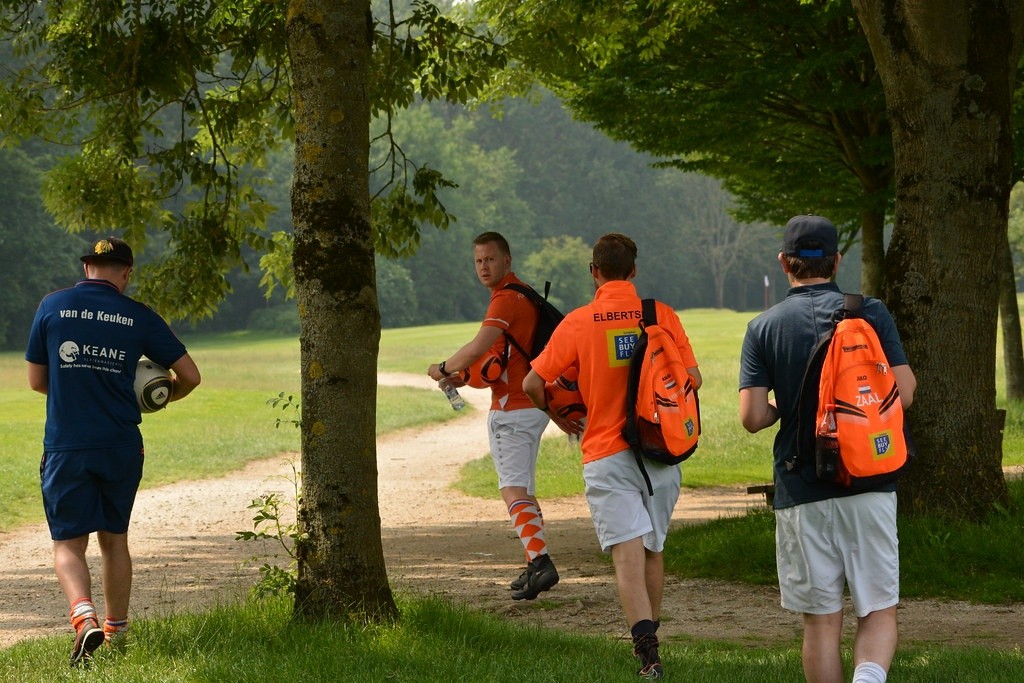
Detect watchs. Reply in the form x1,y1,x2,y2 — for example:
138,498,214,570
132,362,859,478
439,361,451,376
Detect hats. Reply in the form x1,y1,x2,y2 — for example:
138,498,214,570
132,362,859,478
80,236,133,267
779,214,838,257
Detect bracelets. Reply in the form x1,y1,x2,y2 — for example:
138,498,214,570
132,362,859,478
538,406,549,412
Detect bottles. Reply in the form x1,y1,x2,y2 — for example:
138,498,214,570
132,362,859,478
438,378,465,410
819,404,839,448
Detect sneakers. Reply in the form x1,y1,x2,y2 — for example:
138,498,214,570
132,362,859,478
633,650,638,656
510,553,559,600
637,663,663,680
510,568,529,590
103,622,127,660
69,619,104,667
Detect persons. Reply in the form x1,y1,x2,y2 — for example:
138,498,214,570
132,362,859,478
522,233,702,683
26,237,202,667
427,231,551,601
738,214,917,683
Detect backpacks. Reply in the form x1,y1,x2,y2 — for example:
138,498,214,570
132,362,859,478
501,281,565,369
622,299,701,495
783,293,914,497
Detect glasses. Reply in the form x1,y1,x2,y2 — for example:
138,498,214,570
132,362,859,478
590,262,593,273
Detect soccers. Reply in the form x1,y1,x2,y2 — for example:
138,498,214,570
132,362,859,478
543,366,586,421
458,347,502,390
133,361,172,415
